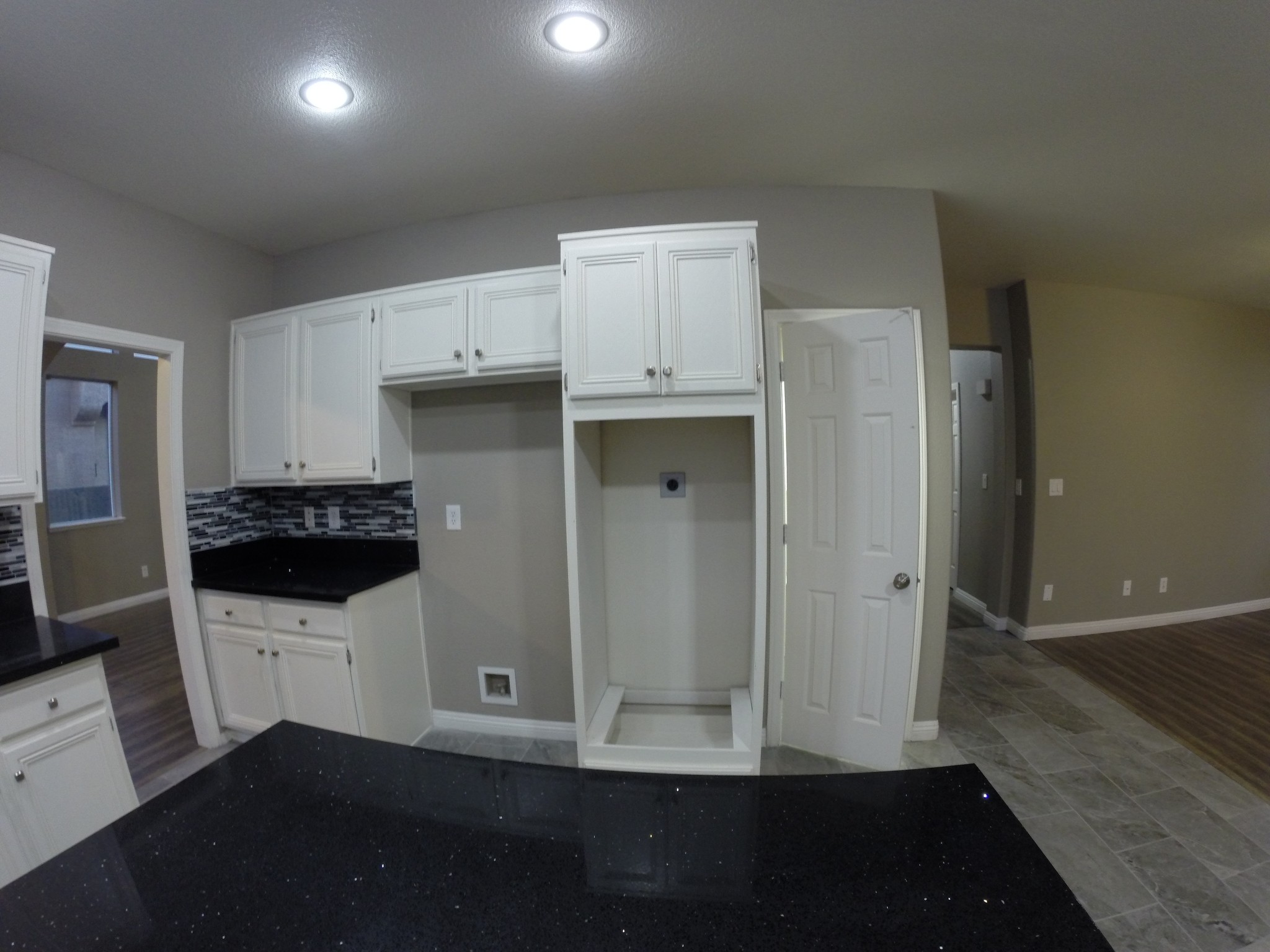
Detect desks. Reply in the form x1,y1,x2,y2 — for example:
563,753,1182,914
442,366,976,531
0,719,1113,952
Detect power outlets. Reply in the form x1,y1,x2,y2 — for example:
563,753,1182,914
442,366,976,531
328,506,340,529
445,505,461,532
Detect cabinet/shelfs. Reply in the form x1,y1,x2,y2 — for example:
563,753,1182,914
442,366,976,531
281,722,756,899
0,241,48,499
203,570,431,745
233,297,412,485
559,240,758,399
378,280,564,393
0,654,142,887
